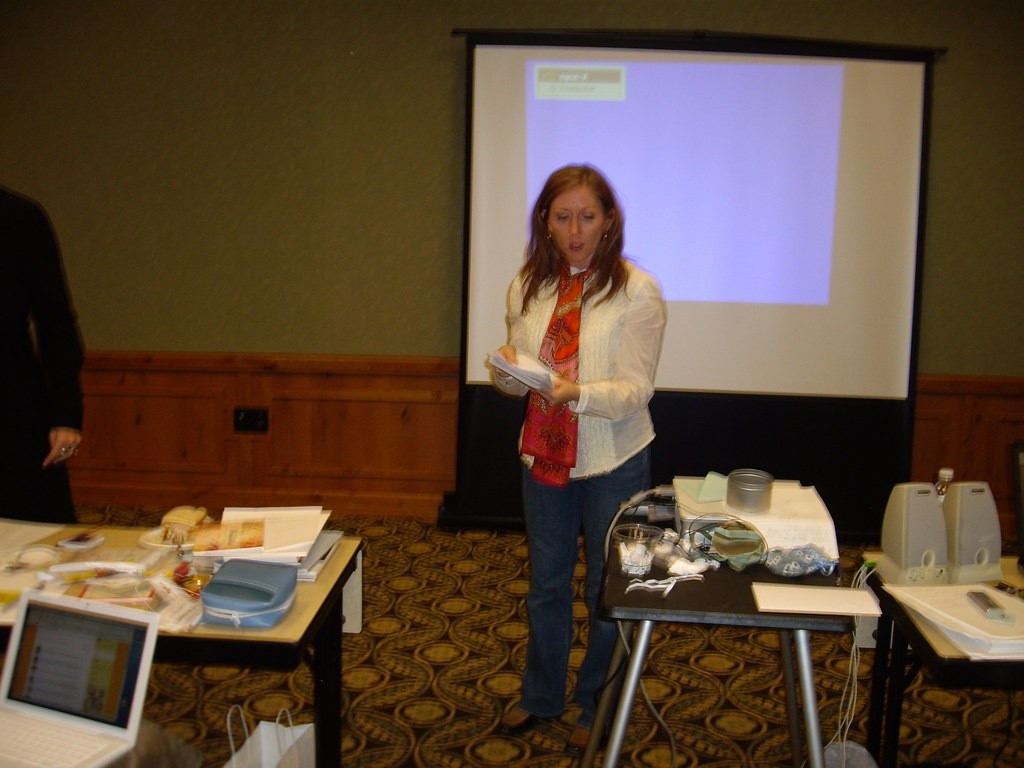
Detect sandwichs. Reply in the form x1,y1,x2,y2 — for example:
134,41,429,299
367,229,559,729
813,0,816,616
160,505,207,544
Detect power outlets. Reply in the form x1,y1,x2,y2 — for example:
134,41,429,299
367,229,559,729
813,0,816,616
234,405,269,434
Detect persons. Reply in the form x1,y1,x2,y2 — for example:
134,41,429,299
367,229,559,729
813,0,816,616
485,164,666,735
0,187,88,525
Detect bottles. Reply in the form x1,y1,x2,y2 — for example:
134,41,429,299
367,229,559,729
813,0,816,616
933,467,954,502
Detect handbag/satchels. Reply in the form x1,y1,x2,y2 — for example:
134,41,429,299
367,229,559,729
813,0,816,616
222,704,315,768
200,558,297,629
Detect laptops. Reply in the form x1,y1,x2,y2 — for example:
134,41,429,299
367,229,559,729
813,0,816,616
0,587,159,768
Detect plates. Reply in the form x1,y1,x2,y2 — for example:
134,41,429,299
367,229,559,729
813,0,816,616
0,543,62,568
139,527,195,552
49,561,148,580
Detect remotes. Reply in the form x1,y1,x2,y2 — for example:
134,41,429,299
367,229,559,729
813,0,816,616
968,591,1003,614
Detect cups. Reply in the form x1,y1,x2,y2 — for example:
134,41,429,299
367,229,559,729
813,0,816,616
726,469,775,514
612,523,664,577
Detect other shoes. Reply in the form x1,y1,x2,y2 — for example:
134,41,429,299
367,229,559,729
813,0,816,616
505,704,532,731
565,725,591,752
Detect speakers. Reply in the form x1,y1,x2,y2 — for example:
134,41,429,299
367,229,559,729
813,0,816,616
878,482,948,586
941,481,1002,584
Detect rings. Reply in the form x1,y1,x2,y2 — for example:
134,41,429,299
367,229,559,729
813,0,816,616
59,447,79,455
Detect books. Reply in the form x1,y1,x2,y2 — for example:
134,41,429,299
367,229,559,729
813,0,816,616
673,481,1024,660
33,506,344,631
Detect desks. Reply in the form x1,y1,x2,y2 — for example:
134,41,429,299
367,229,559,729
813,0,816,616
861,550,1024,768
0,517,362,768
580,543,856,768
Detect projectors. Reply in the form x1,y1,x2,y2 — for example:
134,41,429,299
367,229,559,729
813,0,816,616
672,476,839,560
